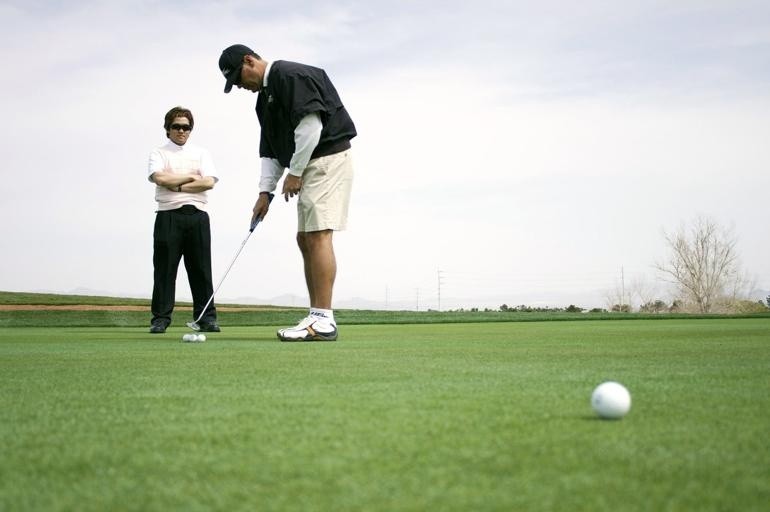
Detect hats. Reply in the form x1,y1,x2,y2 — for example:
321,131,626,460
219,44,254,93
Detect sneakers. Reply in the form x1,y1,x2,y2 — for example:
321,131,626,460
150,320,166,333
277,315,315,338
279,314,338,341
200,320,220,332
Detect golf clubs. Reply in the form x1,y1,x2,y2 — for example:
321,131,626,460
186,193,273,331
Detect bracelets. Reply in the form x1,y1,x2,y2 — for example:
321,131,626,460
258,191,272,198
177,184,183,193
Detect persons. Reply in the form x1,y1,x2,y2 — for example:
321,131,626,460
217,43,358,342
146,103,222,334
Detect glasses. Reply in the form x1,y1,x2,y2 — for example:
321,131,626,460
169,123,191,131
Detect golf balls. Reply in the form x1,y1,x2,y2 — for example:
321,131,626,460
182,334,207,343
592,381,631,418
